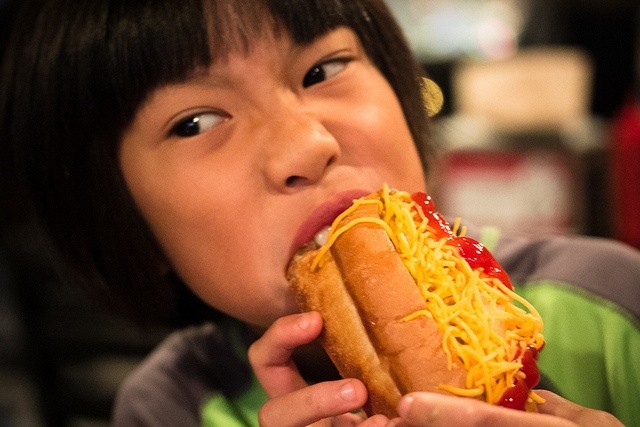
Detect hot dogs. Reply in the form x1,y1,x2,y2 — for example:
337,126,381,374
286,187,545,415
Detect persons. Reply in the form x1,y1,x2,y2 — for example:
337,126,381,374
0,1,640,427
